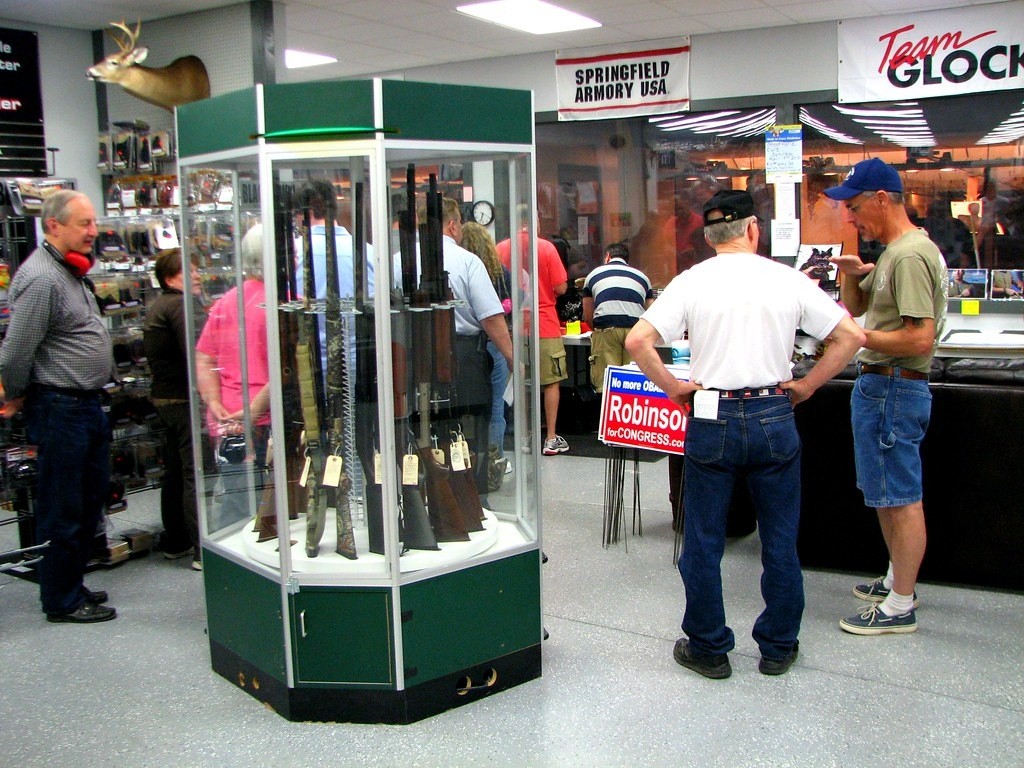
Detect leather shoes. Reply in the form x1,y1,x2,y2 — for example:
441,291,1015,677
42,590,117,624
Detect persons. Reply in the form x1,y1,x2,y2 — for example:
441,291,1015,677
858,182,1012,268
624,189,867,677
148,180,581,571
0,189,117,622
822,157,948,635
582,243,654,394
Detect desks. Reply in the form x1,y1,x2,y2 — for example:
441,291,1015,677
562,336,681,392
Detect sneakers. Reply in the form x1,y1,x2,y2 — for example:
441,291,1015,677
852,576,919,609
543,434,569,456
840,602,918,635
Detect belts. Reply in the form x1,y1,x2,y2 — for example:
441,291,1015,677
861,364,928,380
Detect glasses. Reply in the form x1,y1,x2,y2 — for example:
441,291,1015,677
847,193,875,214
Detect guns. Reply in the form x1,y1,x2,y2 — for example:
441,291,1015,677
324,186,358,560
297,207,328,558
252,185,308,542
391,162,487,550
355,182,384,555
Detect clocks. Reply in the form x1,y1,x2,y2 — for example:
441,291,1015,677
472,200,495,226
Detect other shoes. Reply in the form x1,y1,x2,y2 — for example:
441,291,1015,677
673,637,733,680
505,462,513,474
758,638,799,674
163,545,202,570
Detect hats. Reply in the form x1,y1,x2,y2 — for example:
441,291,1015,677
823,157,903,201
702,188,765,226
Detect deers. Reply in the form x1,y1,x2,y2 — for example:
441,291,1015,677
85,15,211,115
